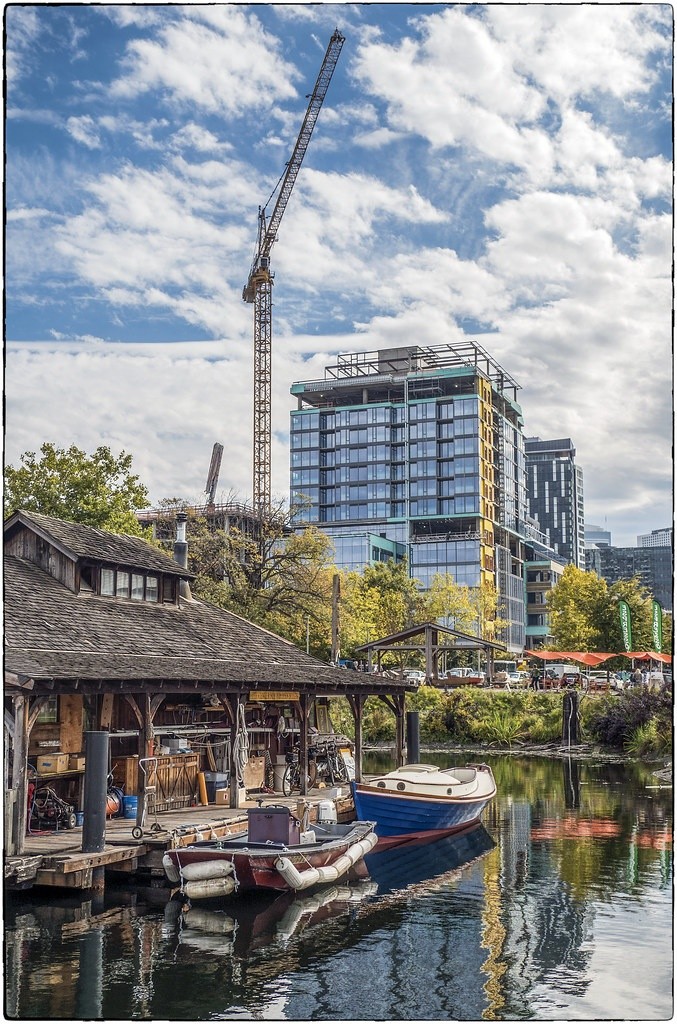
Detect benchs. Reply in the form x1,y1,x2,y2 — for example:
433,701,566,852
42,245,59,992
310,825,354,840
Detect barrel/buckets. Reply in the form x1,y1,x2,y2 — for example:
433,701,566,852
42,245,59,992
123,796,137,819
274,765,291,792
76,812,84,825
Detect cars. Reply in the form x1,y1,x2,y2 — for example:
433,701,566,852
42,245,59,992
402,666,624,691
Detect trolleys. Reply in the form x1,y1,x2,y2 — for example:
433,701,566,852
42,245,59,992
131,758,161,838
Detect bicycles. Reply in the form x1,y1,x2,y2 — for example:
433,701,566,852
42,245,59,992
281,739,348,798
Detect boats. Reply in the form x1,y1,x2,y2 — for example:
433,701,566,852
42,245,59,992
350,761,497,844
159,803,376,898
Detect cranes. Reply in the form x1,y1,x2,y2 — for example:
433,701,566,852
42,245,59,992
241,28,351,631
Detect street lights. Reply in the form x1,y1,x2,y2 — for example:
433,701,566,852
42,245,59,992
543,633,555,692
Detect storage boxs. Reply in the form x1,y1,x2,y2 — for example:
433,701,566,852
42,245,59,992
163,739,187,749
205,771,228,801
70,756,86,770
37,753,70,773
216,788,245,804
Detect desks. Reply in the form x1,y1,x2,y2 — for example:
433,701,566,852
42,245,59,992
244,757,265,793
28,769,85,812
309,747,354,782
